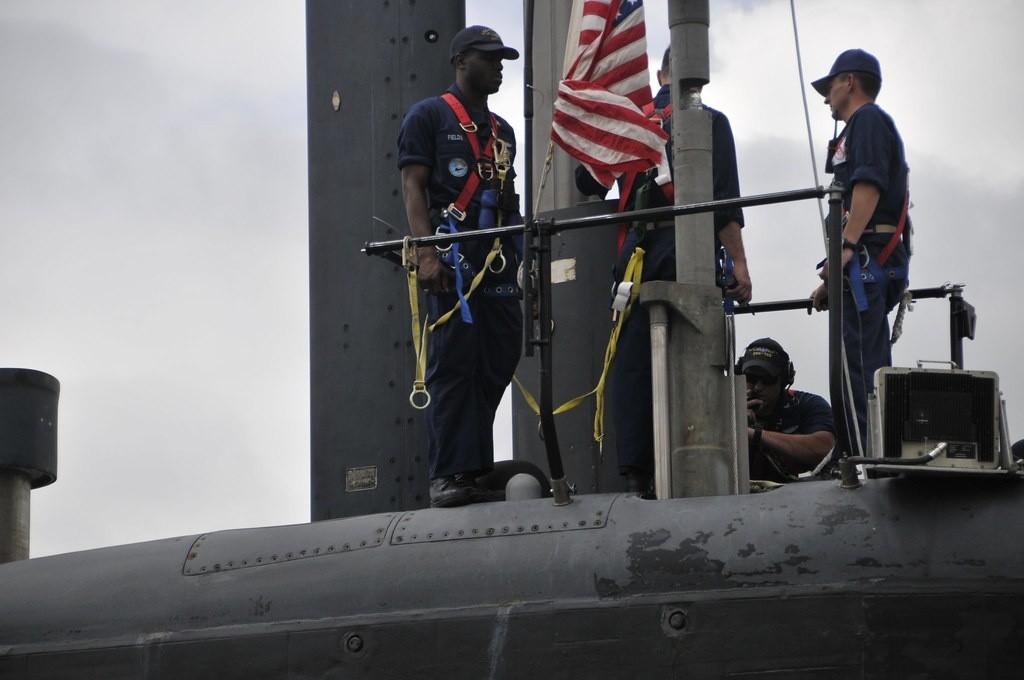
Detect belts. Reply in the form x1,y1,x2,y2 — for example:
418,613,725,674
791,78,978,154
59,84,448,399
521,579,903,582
633,220,677,230
864,224,897,234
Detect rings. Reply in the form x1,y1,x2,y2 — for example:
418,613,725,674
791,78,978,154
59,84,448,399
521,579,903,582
423,289,429,295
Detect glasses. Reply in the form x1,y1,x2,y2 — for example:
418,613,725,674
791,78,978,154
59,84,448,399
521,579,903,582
744,373,781,386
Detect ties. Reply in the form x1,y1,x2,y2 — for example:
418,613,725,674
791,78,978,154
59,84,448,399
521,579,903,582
811,50,881,97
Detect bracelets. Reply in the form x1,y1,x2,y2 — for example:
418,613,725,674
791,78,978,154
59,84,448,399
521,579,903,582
843,237,859,252
754,426,762,447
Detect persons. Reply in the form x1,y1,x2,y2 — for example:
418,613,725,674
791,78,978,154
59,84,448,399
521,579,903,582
613,41,754,494
736,337,837,483
395,25,523,508
809,48,911,480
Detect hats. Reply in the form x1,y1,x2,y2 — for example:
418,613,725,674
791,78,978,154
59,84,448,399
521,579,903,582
451,25,520,60
742,337,788,376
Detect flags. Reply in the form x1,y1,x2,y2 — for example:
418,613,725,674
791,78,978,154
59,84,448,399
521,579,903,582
549,0,675,260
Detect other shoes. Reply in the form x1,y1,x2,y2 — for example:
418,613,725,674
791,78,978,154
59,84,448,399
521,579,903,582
628,476,653,492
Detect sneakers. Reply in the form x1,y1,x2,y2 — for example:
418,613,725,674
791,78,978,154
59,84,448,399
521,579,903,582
456,474,507,502
429,476,471,506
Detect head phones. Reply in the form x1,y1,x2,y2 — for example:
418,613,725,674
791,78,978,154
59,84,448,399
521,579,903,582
737,356,795,385
